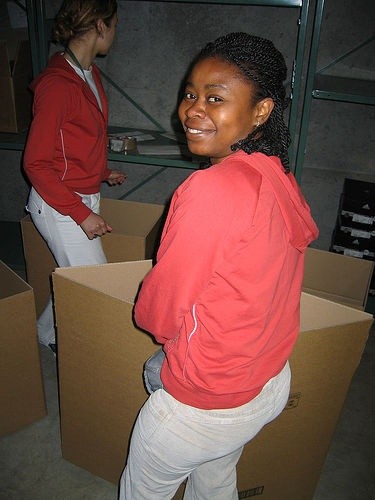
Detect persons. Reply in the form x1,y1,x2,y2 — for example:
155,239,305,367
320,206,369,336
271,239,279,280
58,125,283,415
119,32,320,500
21,0,128,357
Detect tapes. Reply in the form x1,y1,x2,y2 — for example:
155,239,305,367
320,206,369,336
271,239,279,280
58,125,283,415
117,135,137,151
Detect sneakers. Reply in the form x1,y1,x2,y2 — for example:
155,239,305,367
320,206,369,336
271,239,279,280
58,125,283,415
37,328,57,355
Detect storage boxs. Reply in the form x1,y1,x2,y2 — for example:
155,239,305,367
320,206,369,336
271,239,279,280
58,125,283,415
305,247,375,311
0,28,51,135
21,197,165,322
0,260,48,441
51,258,375,500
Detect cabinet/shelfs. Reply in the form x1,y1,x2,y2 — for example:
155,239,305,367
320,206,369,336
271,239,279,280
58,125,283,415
0,0,375,270
